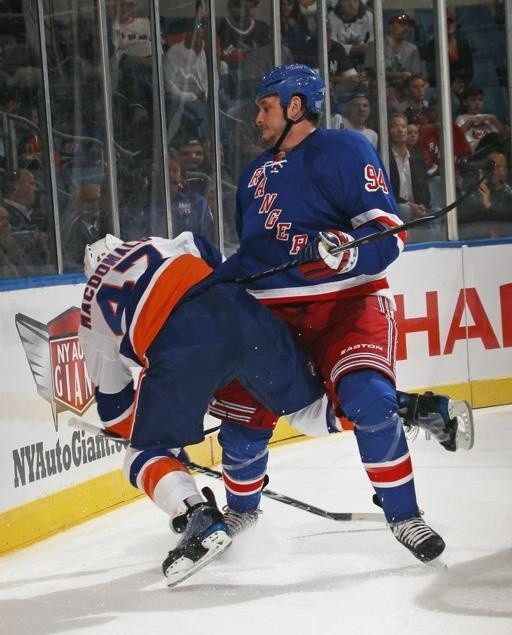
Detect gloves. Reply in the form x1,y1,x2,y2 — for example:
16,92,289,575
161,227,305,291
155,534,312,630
293,227,360,279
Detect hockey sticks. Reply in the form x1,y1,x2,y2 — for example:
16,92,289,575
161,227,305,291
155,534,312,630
68,417,387,522
235,166,498,283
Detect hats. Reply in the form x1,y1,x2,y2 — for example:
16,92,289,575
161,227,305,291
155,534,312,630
387,12,414,26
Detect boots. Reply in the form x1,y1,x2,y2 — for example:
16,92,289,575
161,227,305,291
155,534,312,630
388,513,446,564
396,389,457,453
162,486,229,578
222,474,270,537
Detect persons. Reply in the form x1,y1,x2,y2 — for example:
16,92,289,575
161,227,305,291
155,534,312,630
78,232,458,578
217,63,446,562
1,0,512,280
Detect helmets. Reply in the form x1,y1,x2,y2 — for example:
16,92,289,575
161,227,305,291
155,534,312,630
254,61,325,119
83,232,125,280
